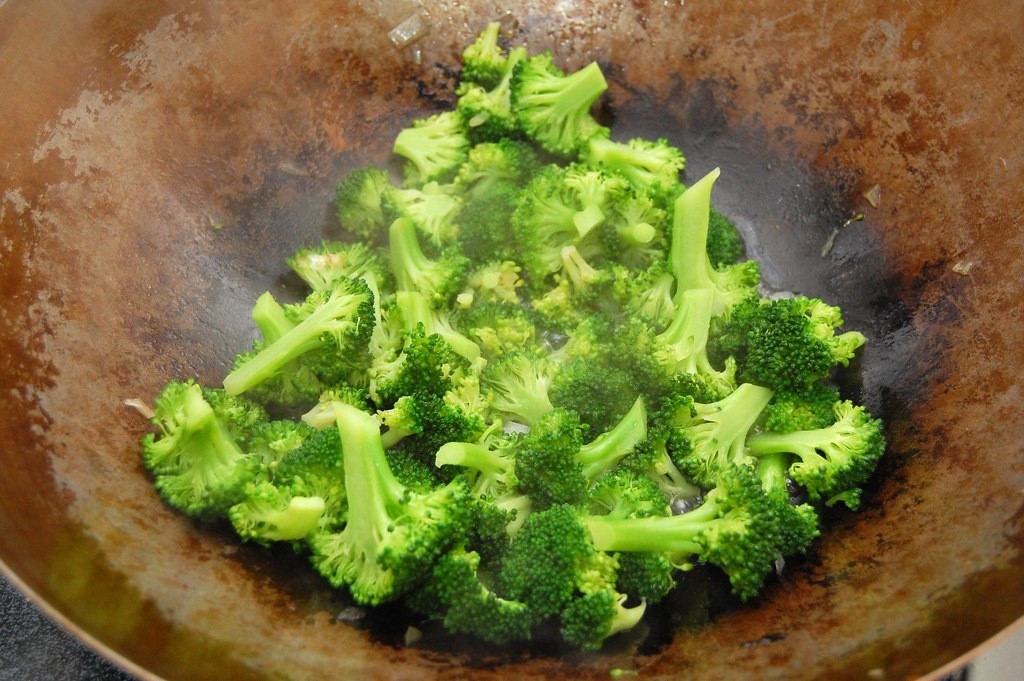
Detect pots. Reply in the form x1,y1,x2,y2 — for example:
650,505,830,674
0,0,1024,681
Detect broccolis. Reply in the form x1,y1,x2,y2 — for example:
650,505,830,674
140,20,887,652
